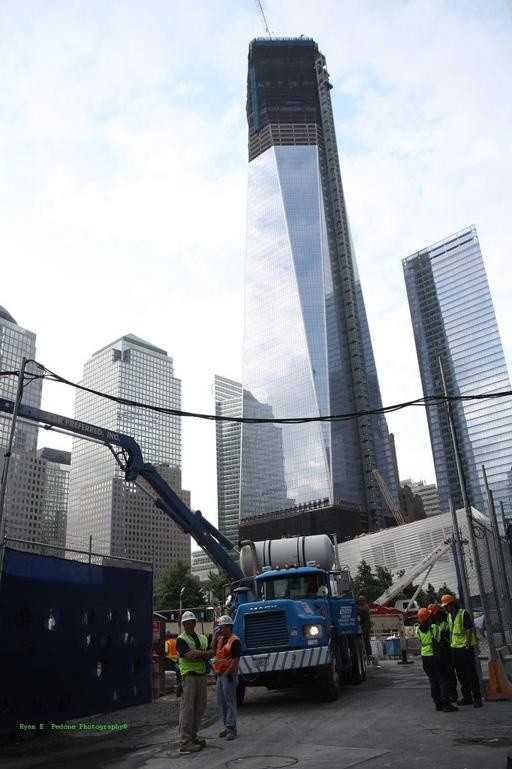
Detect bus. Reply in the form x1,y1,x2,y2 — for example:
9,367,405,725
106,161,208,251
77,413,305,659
152,606,218,646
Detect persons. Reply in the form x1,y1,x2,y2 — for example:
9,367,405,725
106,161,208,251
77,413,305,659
416,606,459,713
427,602,459,704
213,615,243,741
176,610,215,753
440,592,485,707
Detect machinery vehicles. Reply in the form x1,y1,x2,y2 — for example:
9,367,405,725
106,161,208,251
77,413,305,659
0,399,373,705
365,537,470,632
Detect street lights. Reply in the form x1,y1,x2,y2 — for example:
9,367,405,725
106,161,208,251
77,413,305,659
177,585,185,637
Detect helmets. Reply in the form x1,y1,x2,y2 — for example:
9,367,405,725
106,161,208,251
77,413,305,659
418,595,453,622
218,615,234,625
181,611,197,624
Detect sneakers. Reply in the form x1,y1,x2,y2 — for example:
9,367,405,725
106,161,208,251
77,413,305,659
180,729,237,752
435,696,482,712
